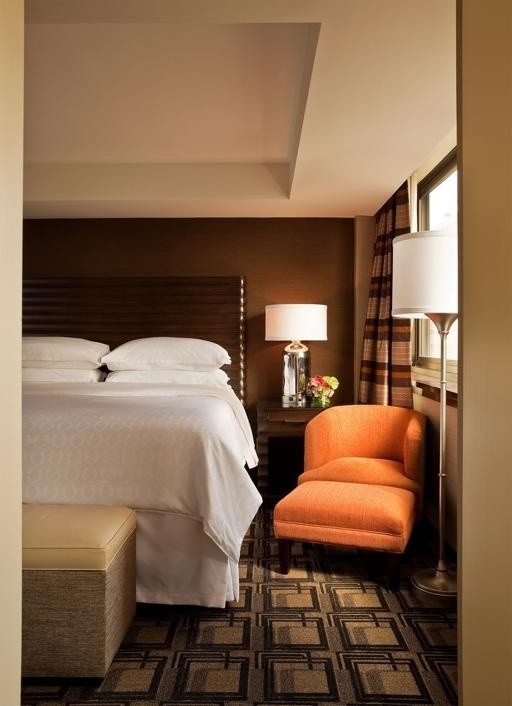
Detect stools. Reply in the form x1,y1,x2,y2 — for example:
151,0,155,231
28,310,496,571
22,504,137,677
274,481,416,591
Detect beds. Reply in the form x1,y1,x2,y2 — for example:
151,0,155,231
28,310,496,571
23,276,247,613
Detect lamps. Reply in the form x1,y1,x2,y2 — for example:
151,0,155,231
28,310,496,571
264,304,328,404
391,229,458,598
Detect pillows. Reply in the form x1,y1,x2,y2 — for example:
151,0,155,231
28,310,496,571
23,369,107,382
105,369,232,390
23,336,110,369
101,337,232,371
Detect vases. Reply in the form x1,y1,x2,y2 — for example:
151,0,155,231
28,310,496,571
311,396,333,408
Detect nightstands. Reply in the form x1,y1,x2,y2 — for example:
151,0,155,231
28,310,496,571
256,397,335,507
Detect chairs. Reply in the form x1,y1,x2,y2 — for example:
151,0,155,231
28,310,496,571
298,405,428,550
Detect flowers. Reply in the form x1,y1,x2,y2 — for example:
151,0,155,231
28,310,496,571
305,375,340,398
